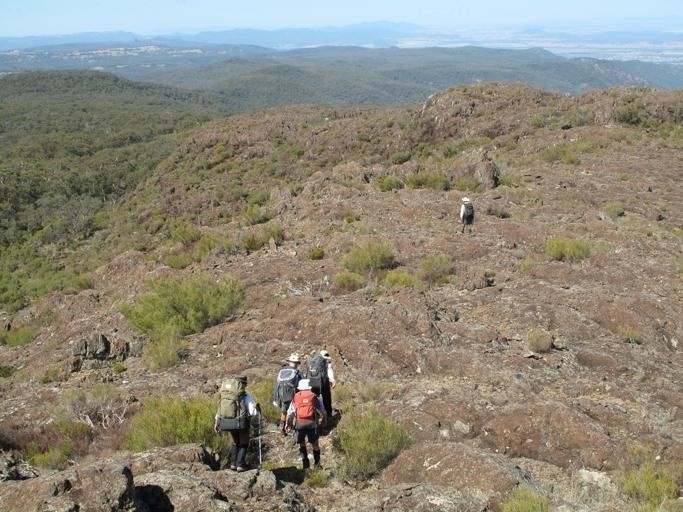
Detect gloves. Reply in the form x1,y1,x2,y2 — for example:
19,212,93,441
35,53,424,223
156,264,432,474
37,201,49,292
293,391,323,430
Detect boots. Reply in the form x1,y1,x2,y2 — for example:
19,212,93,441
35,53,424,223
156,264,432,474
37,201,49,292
298,446,310,469
312,449,323,470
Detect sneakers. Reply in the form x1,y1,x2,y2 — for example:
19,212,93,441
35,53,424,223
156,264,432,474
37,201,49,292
229,464,250,472
278,424,293,438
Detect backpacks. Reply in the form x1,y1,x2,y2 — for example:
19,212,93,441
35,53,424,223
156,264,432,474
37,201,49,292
218,379,248,431
278,369,297,404
463,203,474,216
306,356,329,390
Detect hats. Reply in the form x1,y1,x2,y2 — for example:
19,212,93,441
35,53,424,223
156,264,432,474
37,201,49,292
234,375,247,384
296,378,312,391
461,197,469,202
319,350,331,360
286,355,301,365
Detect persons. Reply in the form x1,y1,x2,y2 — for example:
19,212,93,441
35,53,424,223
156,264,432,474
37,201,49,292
283,378,328,470
304,349,339,418
271,353,305,437
459,195,472,235
214,373,260,471
